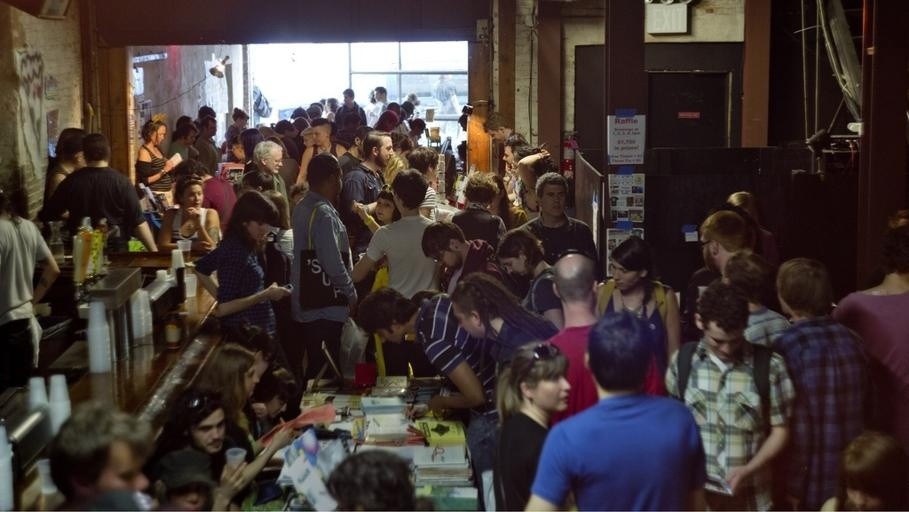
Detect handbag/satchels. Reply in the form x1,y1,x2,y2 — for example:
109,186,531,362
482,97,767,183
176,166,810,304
299,248,349,309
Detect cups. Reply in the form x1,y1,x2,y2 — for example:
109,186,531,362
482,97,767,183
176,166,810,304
164,327,184,349
0,418,17,511
155,248,197,300
86,301,112,376
225,448,247,472
177,240,191,259
131,291,153,340
27,373,73,435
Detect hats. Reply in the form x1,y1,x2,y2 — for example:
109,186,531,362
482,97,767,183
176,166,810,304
236,190,280,227
160,449,215,492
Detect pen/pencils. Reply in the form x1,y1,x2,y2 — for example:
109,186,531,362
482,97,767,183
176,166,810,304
279,417,286,426
407,390,418,421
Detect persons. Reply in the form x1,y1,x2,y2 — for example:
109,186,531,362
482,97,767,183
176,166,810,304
2,81,797,511
835,208,909,449
770,258,881,512
820,431,909,512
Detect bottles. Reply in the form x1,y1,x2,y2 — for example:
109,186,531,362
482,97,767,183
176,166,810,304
96,217,108,248
81,216,93,232
48,220,67,265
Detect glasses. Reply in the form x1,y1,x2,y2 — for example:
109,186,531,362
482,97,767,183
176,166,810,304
381,184,394,195
187,390,223,408
521,342,560,381
